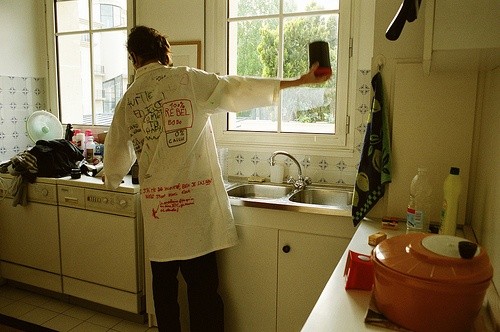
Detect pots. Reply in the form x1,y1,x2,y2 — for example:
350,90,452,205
368,233,494,332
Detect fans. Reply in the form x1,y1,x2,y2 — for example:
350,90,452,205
26,110,64,144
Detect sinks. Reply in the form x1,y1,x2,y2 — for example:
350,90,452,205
226,182,297,201
288,186,354,207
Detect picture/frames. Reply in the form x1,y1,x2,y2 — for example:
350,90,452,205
170,41,201,69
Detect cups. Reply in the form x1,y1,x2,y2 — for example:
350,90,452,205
309,41,332,76
271,165,284,184
216,146,230,187
71,168,81,179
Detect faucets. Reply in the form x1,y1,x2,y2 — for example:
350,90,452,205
269,150,303,189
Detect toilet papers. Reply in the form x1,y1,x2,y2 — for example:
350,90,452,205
270,164,284,183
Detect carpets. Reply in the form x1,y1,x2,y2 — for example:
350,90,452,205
0,314,60,332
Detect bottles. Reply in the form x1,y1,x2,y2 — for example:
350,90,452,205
65,124,102,166
406,167,433,232
438,166,462,236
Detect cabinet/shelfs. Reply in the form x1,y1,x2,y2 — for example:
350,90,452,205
145,225,353,332
424,0,500,76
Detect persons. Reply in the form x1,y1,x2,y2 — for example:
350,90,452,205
101,27,332,332
